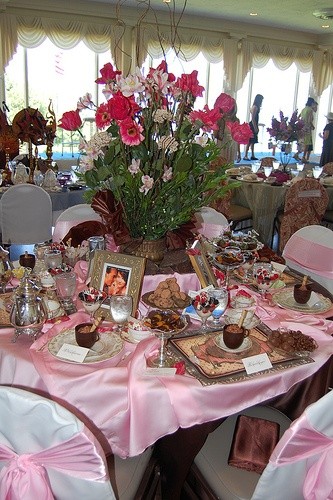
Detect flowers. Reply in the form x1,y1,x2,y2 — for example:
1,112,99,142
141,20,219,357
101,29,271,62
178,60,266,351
58,60,254,241
267,110,308,174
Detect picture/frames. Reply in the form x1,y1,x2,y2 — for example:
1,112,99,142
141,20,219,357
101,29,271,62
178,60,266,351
88,249,146,318
188,239,218,289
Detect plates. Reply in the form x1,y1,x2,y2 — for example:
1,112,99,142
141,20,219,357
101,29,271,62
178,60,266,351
213,332,252,352
225,167,279,184
272,287,331,313
283,175,333,188
0,185,64,194
47,329,123,364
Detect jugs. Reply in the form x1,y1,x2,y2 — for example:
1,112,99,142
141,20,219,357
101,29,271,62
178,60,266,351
44,168,58,186
10,269,48,343
11,162,31,184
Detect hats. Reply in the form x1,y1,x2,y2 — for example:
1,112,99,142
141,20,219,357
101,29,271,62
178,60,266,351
324,112,333,120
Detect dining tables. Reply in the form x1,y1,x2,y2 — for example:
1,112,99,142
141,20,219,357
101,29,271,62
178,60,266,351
229,170,332,248
0,273,333,500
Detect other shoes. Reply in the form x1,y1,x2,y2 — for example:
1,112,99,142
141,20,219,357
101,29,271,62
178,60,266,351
251,157,258,160
243,157,251,161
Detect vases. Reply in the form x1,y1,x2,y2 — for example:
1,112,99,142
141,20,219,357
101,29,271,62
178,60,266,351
131,241,163,264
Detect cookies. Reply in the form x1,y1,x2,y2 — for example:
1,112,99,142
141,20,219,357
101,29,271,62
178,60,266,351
148,278,187,307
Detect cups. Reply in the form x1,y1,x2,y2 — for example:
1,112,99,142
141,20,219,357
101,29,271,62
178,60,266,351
223,324,250,349
87,236,104,255
74,322,100,347
19,254,35,270
294,284,311,304
34,245,63,268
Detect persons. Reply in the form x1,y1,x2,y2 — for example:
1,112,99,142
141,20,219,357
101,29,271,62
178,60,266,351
231,104,241,163
243,94,265,161
319,112,333,167
307,101,318,160
102,266,129,297
292,97,315,163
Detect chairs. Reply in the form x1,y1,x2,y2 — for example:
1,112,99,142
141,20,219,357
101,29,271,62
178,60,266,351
229,204,254,234
281,225,333,295
53,204,107,245
0,384,119,500
0,183,52,260
270,177,329,257
259,157,281,168
191,206,228,241
183,386,333,500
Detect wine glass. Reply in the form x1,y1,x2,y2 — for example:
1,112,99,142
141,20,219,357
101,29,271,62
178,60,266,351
54,272,76,310
190,245,276,333
109,296,133,330
143,313,188,369
78,293,104,323
